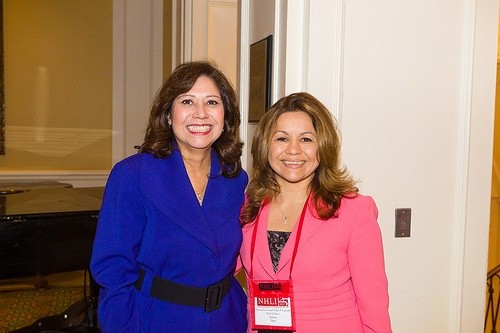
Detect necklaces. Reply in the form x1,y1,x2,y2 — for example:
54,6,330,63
184,162,210,204
275,192,308,224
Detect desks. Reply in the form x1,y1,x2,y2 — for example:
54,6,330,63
0,186,115,333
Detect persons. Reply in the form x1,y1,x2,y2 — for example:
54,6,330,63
90,61,248,333
234,92,392,333
